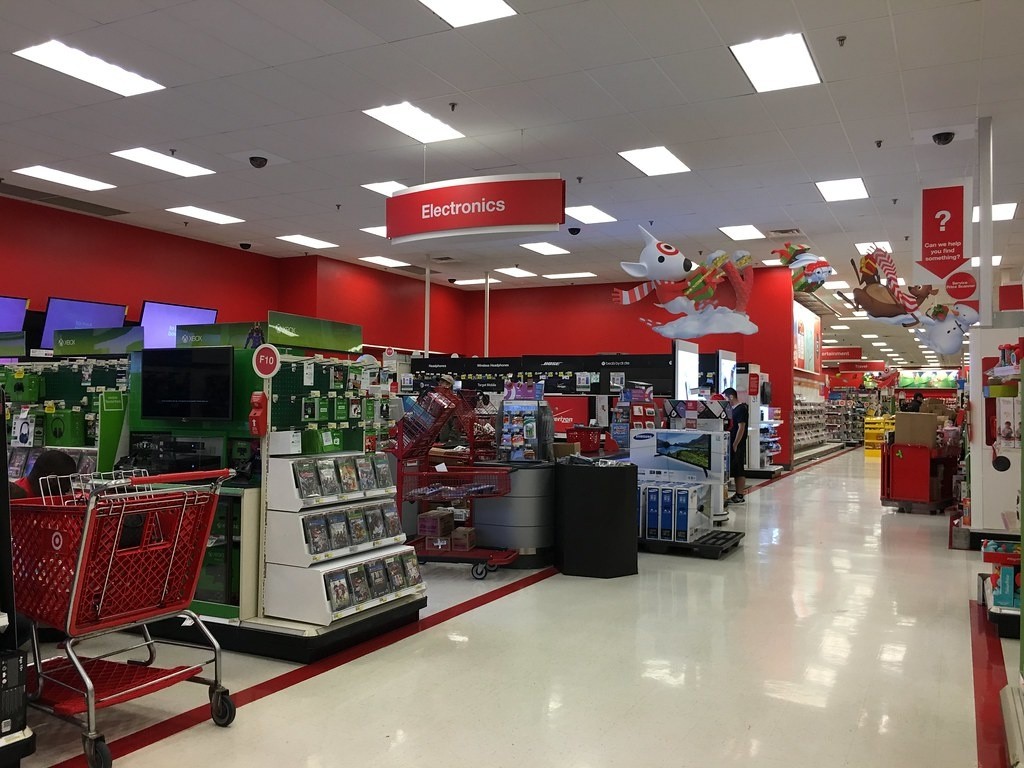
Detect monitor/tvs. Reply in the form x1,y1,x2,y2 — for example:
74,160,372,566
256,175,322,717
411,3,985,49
140,344,234,423
39,297,129,350
654,433,711,479
138,300,219,349
763,382,771,404
0,296,30,332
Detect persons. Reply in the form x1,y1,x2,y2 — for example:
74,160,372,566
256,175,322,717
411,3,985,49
1002,421,1013,440
904,392,924,412
1,449,77,648
435,374,462,443
701,382,726,400
723,388,748,504
1016,422,1022,441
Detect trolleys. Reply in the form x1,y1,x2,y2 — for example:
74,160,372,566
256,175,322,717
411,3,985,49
390,387,521,578
10,469,236,768
879,440,959,515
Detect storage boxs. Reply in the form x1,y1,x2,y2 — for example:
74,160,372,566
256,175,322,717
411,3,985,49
638,480,713,543
992,563,1015,607
893,398,954,447
416,507,476,553
0,371,87,448
952,458,970,525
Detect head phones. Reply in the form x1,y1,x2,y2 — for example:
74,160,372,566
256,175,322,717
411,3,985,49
19,422,29,444
51,418,65,438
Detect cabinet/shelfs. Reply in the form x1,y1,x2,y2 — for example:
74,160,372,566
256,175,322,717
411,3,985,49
793,384,897,466
882,439,960,514
967,322,1024,768
0,310,428,665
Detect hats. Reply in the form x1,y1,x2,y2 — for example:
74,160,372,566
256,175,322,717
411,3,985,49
441,374,455,385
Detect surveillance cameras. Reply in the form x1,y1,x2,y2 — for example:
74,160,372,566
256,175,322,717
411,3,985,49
239,243,251,249
568,228,581,235
249,157,267,169
932,132,954,145
448,279,456,283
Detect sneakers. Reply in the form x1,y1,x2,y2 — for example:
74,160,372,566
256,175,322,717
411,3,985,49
725,494,746,505
